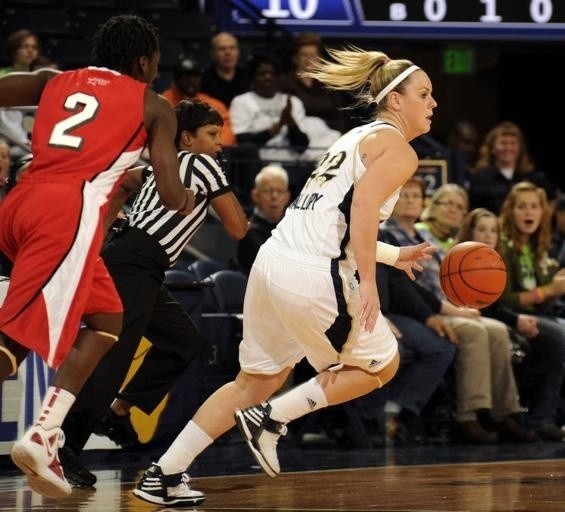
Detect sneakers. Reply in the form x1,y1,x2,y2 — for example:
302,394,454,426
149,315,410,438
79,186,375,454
451,417,497,444
10,419,75,503
233,399,288,479
54,442,98,490
527,412,564,443
131,460,206,508
494,418,535,444
91,406,140,447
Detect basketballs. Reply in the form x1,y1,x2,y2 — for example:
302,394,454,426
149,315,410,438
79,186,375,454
439,240,506,309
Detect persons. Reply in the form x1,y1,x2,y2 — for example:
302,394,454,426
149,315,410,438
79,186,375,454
56,95,249,490
2,10,197,501
134,42,439,503
1,22,565,448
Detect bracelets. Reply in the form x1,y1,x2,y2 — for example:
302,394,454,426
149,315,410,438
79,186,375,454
374,239,401,269
179,190,189,213
